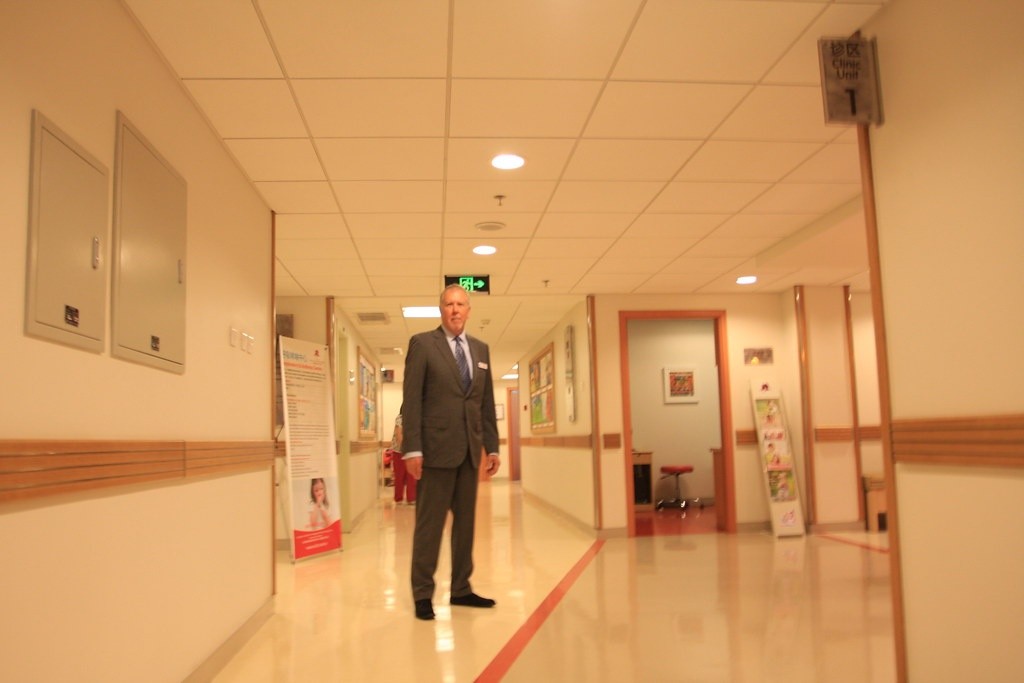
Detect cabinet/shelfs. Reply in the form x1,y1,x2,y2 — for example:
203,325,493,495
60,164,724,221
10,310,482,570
633,452,656,512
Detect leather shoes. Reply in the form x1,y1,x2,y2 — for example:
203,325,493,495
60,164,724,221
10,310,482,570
450,594,496,608
415,599,435,620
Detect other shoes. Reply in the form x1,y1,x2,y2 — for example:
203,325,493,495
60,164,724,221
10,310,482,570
407,501,417,505
395,501,403,505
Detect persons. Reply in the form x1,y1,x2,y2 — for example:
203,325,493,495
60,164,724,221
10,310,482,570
400,282,502,619
307,479,334,531
391,403,418,505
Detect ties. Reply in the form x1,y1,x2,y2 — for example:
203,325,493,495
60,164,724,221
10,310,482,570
451,336,471,391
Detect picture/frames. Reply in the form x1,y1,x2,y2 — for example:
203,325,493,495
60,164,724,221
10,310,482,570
664,368,699,404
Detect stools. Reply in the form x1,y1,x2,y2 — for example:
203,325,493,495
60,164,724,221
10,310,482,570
656,465,705,510
